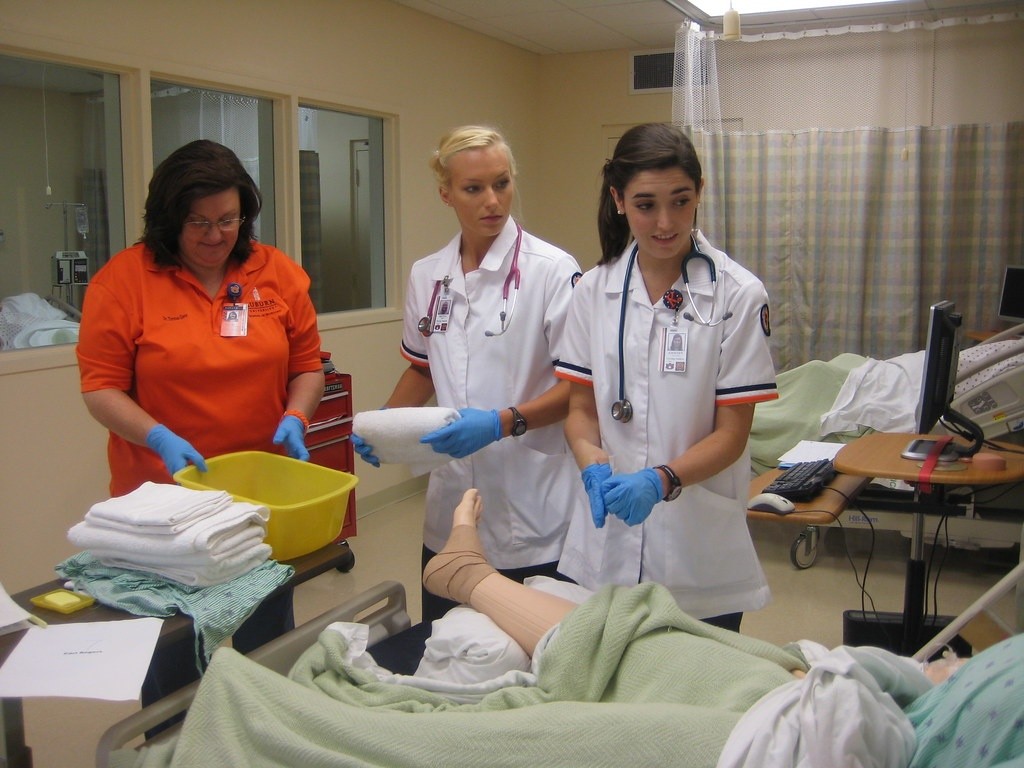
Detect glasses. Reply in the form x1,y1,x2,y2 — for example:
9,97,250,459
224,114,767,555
183,217,245,231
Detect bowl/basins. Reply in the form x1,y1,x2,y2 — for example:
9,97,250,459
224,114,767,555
174,449,354,561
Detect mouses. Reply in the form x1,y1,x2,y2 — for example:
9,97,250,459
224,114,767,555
747,493,795,515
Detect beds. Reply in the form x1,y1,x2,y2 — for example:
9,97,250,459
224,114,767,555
94,563,1024,768
790,323,1024,570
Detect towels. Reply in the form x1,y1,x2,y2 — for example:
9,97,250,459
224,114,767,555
65,479,273,594
819,348,924,437
713,638,934,766
352,407,464,465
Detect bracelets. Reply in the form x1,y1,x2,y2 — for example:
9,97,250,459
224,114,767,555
284,409,309,427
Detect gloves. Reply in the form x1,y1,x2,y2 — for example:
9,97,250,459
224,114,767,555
348,407,393,468
581,463,614,529
601,469,663,528
145,424,208,484
273,415,311,463
419,406,501,458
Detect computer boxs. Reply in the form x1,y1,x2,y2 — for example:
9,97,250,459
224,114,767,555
842,610,972,663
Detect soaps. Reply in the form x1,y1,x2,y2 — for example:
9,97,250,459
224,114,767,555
44,593,80,608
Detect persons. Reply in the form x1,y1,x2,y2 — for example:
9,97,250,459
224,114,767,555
227,312,237,320
351,124,582,624
422,484,1024,768
551,120,779,638
76,140,326,740
670,334,683,351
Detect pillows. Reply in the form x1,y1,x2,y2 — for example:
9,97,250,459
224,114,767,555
414,576,596,685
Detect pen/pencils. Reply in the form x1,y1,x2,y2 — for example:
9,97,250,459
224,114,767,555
29,614,48,629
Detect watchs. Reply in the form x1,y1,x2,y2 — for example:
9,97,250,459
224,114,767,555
653,464,684,503
508,407,528,437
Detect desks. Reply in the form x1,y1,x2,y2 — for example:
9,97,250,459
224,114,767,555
965,330,1002,342
745,434,874,525
833,433,1024,663
0,543,353,768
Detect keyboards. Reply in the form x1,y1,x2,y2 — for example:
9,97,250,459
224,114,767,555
760,459,840,503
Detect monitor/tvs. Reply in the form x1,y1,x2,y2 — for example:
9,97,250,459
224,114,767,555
997,265,1024,323
901,300,984,462
